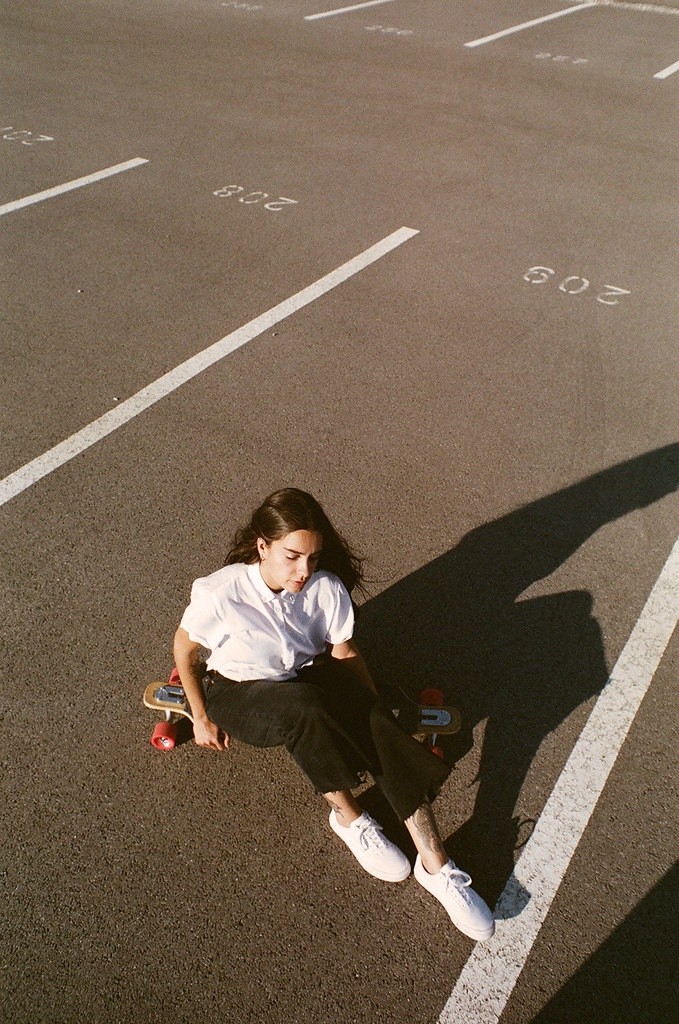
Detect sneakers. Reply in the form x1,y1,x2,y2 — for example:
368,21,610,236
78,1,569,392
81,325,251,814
414,852,495,942
329,807,411,883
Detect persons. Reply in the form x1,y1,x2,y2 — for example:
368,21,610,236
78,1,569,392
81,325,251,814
172,487,496,941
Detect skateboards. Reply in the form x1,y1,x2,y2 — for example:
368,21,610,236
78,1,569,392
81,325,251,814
142,666,464,760
